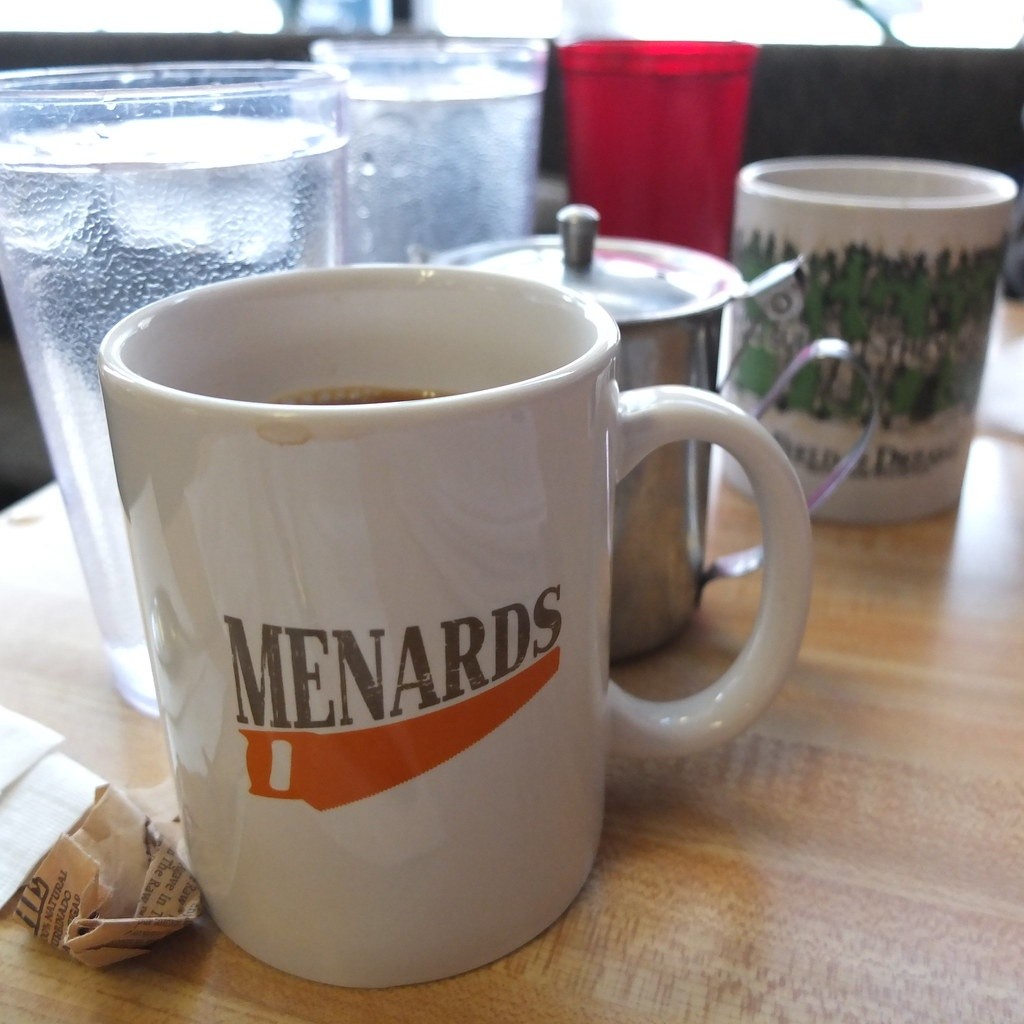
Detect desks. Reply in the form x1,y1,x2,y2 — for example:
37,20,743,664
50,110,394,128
0,297,1023,1024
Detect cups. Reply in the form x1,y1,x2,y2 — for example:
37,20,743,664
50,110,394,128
96,263,813,994
3,64,348,719
420,205,882,662
314,39,549,258
716,156,1018,519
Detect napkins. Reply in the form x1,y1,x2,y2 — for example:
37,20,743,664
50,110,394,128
0,704,108,916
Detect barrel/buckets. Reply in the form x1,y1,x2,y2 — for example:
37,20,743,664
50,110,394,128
560,40,758,256
